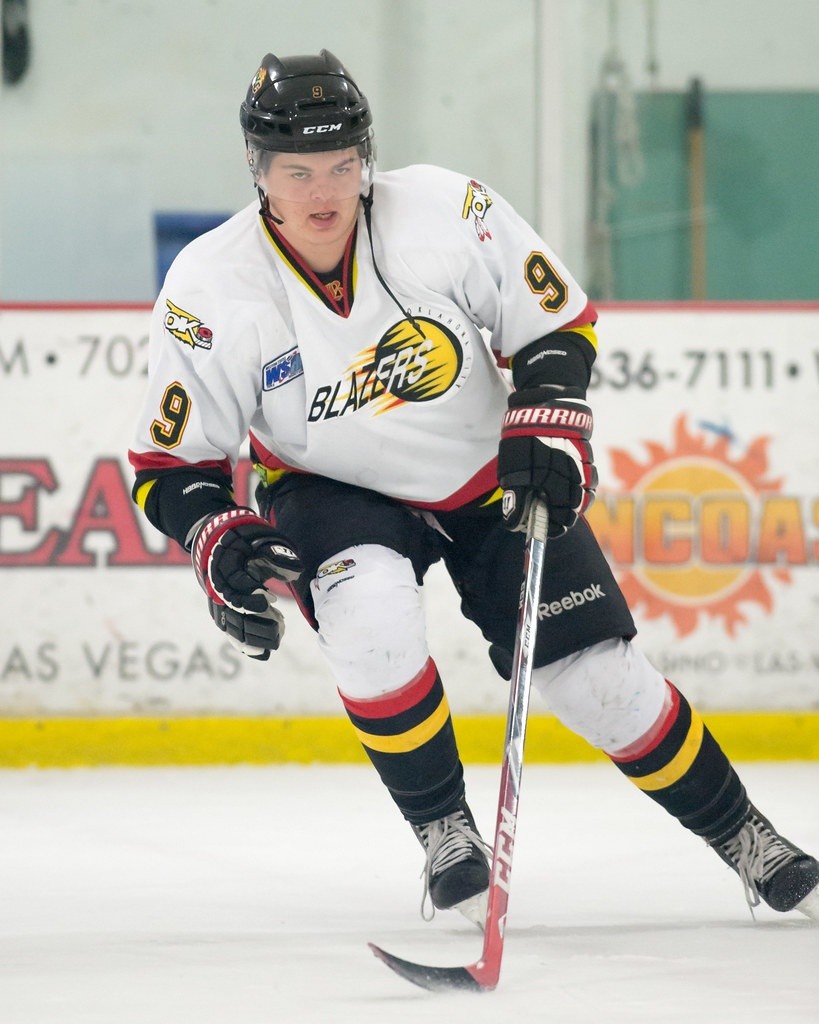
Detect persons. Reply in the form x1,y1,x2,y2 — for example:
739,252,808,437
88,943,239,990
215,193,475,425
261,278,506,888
130,46,819,931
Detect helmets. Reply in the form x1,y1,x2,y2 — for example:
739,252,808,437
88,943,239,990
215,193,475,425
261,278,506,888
240,48,373,152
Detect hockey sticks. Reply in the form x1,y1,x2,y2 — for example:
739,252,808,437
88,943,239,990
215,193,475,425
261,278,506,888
366,494,550,1000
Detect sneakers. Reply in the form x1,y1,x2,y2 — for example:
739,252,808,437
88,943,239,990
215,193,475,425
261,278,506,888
710,804,818,926
411,800,487,933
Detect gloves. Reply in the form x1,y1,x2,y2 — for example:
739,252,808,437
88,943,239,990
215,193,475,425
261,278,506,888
498,389,599,539
191,505,304,661
